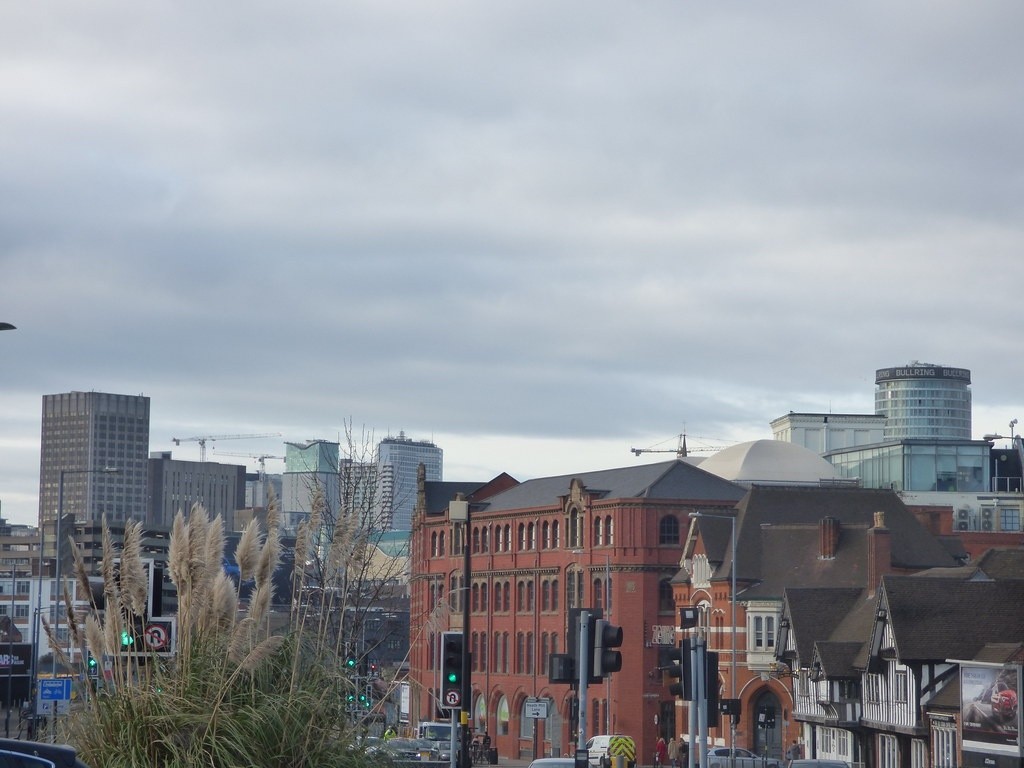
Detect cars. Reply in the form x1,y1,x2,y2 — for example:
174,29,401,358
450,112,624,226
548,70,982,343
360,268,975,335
347,736,460,761
788,760,850,768
528,756,595,768
0,738,92,768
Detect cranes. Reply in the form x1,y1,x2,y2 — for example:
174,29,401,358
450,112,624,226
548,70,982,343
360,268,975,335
630,432,733,456
173,434,282,461
214,452,285,481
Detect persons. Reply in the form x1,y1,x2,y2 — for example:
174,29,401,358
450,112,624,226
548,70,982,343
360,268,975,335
668,738,680,768
27,719,32,740
655,738,666,768
787,740,801,759
679,738,686,759
384,724,398,741
466,730,491,750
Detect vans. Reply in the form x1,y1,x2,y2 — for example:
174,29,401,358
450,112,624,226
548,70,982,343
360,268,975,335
585,735,636,768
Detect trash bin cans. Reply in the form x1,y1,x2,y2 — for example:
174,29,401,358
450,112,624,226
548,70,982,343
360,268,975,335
616,755,624,768
680,754,687,768
490,747,498,765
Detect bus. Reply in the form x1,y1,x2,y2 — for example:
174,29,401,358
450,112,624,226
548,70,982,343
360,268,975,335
418,721,461,747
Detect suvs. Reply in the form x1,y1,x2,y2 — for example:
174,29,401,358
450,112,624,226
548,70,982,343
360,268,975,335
991,683,1018,718
706,747,781,768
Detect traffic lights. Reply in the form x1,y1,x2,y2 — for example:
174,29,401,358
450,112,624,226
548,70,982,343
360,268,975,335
346,679,356,703
86,655,97,676
441,631,463,710
107,558,176,658
346,642,356,671
359,680,372,708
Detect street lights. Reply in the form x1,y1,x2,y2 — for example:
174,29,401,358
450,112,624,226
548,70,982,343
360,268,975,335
573,550,610,735
50,467,119,744
668,640,687,700
593,619,623,677
28,518,87,711
362,619,381,662
6,564,50,739
687,512,735,767
27,604,80,738
